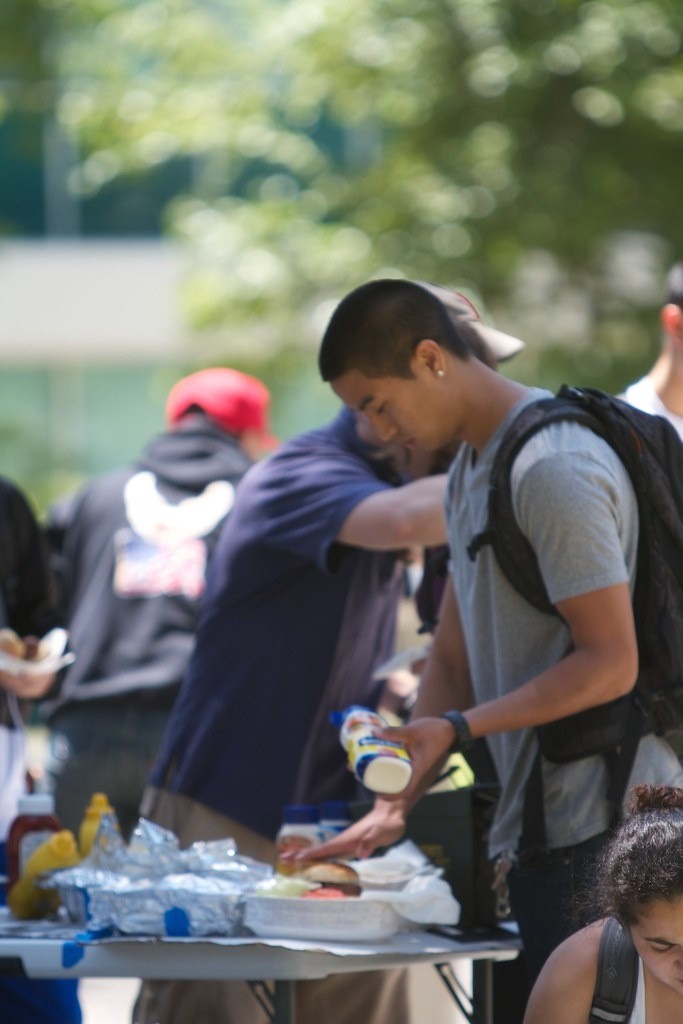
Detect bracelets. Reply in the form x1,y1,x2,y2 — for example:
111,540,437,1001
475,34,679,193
441,712,475,751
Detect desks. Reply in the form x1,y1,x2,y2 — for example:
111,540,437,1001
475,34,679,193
1,918,523,1024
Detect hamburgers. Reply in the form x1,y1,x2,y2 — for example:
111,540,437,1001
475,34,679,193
301,861,361,899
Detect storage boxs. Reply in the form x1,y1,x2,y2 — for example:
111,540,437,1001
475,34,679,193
345,786,501,942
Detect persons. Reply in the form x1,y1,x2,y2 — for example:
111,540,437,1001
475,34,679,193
524,782,683,1023
2,256,682,1024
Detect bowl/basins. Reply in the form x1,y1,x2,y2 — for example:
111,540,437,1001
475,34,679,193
242,892,404,942
361,863,418,892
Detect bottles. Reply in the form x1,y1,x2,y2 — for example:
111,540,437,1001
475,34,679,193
6,829,79,921
80,792,119,859
8,795,60,889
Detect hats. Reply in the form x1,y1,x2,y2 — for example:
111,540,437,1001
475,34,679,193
166,367,271,436
417,279,524,363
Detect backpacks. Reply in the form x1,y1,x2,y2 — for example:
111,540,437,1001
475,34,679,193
466,382,682,765
